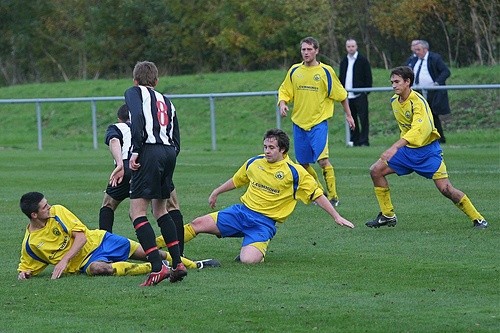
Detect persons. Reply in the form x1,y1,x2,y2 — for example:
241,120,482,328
99,104,184,257
155,129,355,264
125,61,188,286
17,192,203,280
277,38,355,207
365,66,488,227
403,39,451,143
338,39,373,146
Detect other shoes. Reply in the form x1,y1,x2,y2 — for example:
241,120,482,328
329,199,339,206
235,255,240,261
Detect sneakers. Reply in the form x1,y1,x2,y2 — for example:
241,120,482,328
474,219,487,228
162,259,169,266
195,259,221,269
366,211,396,228
139,261,171,286
168,263,187,283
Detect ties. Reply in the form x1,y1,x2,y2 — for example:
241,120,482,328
415,58,424,85
